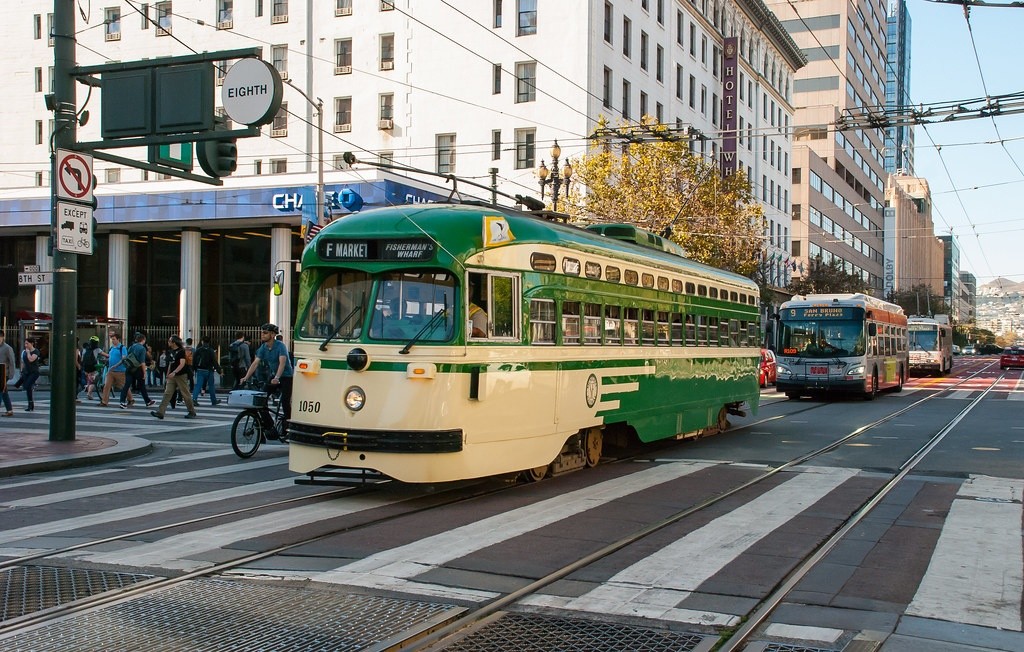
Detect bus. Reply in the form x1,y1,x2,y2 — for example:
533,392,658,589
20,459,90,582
765,293,909,401
906,292,952,378
274,151,762,497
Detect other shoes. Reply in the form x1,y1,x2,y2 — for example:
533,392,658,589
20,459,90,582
212,399,221,405
97,402,108,407
86,393,94,400
126,399,135,408
2,410,13,417
193,402,199,406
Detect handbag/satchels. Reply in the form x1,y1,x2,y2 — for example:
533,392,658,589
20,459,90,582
122,353,141,375
227,390,268,409
153,366,162,378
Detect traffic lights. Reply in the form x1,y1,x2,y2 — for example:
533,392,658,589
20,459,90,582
89,174,98,255
195,126,237,179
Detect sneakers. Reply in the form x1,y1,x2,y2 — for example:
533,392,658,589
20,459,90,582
184,412,196,419
146,400,155,406
151,411,164,419
119,403,128,409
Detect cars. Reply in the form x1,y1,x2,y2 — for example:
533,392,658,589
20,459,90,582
1000,344,1024,371
952,344,1003,356
760,349,776,388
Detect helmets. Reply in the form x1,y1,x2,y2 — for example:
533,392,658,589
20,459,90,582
88,335,100,343
261,323,280,334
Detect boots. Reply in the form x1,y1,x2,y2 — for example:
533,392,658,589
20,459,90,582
25,401,34,411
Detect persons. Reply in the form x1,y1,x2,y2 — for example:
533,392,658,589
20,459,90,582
14,338,41,412
240,323,293,437
0,330,15,416
384,298,407,320
469,283,487,338
74,333,252,420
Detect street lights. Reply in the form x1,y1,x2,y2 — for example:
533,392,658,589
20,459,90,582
538,137,573,223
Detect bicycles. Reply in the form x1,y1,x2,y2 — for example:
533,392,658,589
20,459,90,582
95,357,115,401
230,380,290,460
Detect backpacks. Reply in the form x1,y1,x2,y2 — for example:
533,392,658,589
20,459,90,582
229,341,245,369
184,346,193,367
80,346,99,374
193,345,214,380
